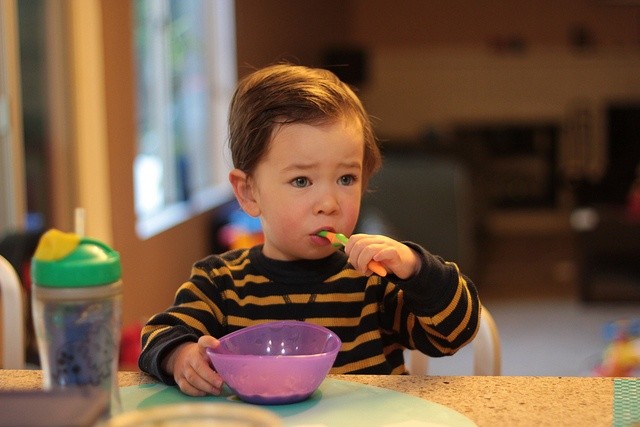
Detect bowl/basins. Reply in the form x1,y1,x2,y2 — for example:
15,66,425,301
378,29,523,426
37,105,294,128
206,323,341,406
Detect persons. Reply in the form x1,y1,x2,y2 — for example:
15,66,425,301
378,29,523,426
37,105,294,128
137,64,480,397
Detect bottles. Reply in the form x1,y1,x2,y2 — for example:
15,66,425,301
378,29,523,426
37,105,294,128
31,207,122,422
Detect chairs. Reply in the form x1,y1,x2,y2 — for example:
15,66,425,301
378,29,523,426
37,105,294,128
407,302,502,377
